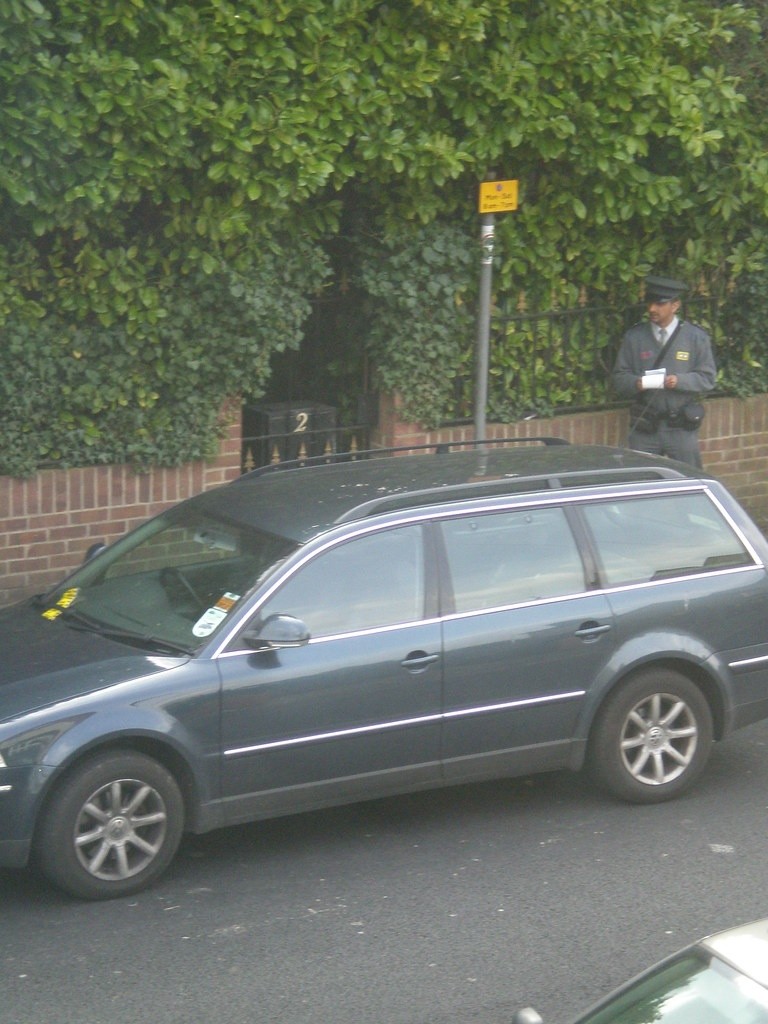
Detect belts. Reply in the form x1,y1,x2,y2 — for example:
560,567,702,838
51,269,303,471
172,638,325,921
638,402,680,421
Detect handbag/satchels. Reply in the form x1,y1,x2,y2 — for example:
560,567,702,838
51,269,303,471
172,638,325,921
629,405,660,435
681,404,704,431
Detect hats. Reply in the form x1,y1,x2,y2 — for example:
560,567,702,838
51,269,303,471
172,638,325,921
644,275,688,303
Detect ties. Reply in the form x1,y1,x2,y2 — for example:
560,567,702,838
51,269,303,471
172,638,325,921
659,330,667,345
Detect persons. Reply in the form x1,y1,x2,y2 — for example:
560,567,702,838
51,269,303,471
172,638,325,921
610,276,716,468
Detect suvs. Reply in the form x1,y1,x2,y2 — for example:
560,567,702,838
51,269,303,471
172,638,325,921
0,435,768,894
